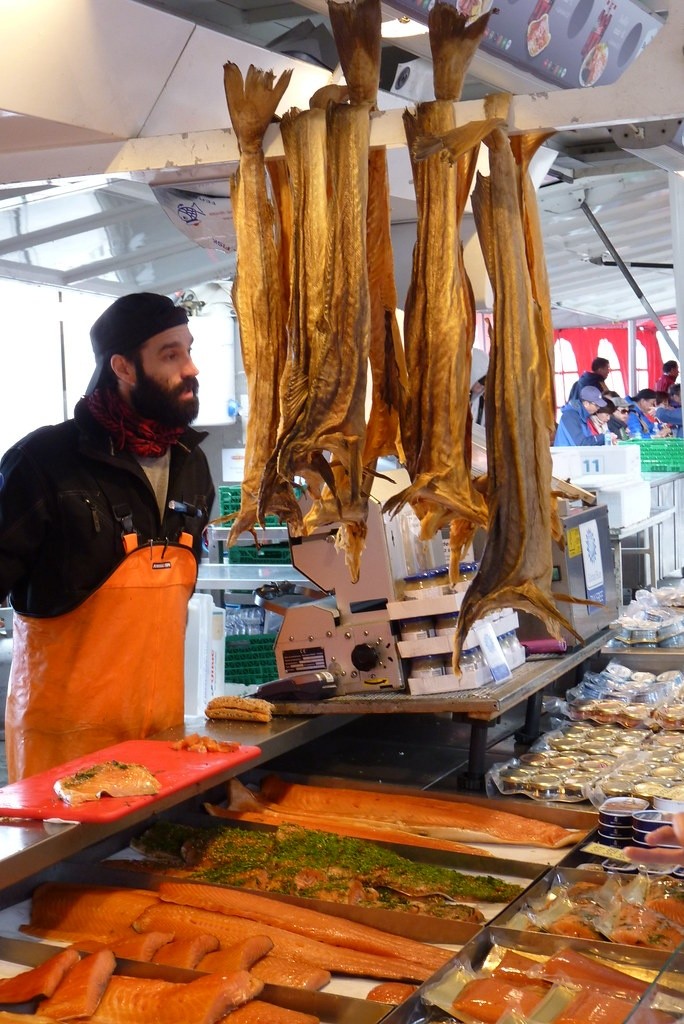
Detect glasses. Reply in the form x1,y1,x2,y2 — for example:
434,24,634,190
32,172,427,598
617,408,631,415
477,376,487,385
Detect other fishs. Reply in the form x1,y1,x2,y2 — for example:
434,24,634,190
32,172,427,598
201,0,599,679
0,761,684,1024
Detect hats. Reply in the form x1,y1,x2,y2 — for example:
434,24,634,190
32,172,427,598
610,397,634,409
580,385,608,408
85,293,189,396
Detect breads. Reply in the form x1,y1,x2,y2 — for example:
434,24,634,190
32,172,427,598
205,696,274,722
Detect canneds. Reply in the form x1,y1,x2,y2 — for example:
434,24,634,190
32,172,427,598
496,609,684,808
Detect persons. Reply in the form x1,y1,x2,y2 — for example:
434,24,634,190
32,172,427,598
0,292,216,781
554,357,683,446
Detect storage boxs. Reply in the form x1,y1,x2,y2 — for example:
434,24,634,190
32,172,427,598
548,436,684,528
219,484,307,691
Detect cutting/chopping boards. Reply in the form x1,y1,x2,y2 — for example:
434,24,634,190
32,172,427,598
0,740,262,822
